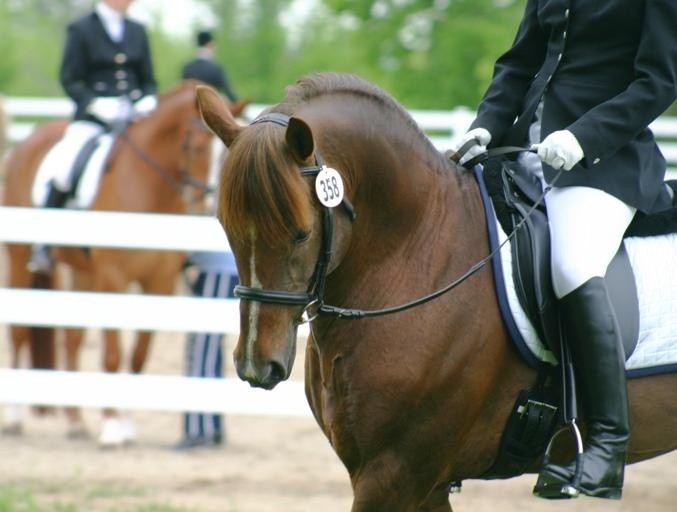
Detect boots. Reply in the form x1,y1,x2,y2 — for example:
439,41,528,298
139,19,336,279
27,184,70,273
544,278,630,499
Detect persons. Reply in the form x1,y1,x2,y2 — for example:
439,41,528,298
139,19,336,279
452,1,677,503
26,0,160,274
168,210,243,453
181,27,250,122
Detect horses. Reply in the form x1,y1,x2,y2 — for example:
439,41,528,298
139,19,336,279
193,69,677,512
0,77,250,450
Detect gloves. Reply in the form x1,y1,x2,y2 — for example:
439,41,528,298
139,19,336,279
94,97,119,120
530,129,585,170
135,96,155,115
456,127,492,164
